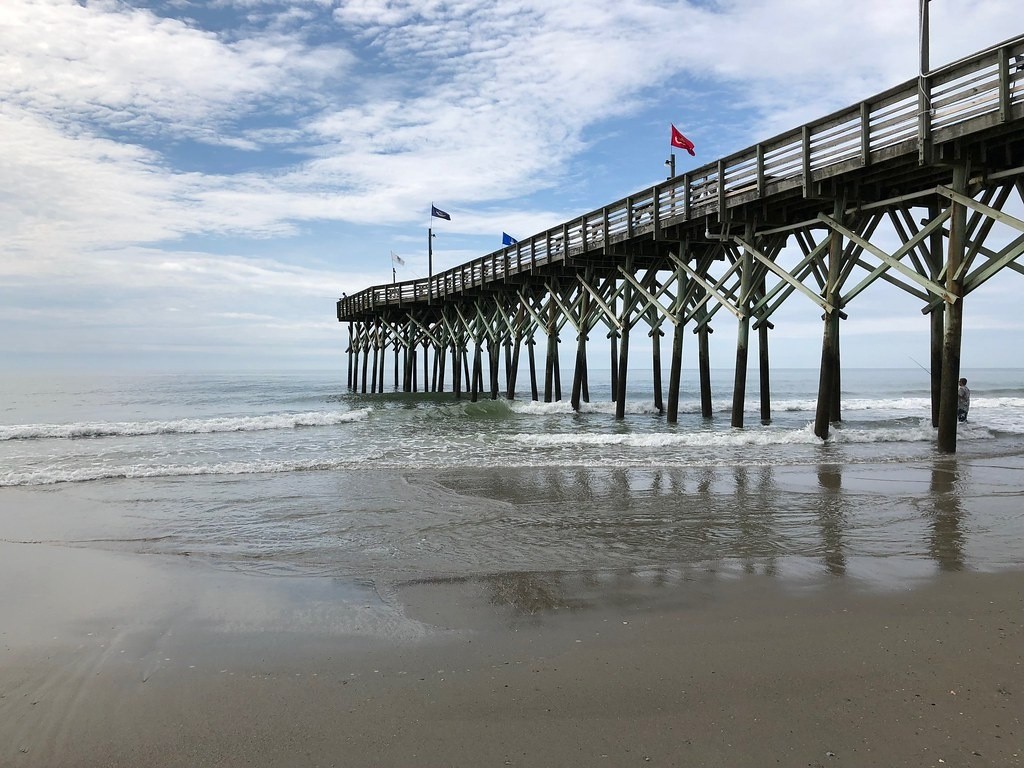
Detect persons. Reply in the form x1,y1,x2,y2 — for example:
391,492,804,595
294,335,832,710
959,377,970,422
339,292,347,301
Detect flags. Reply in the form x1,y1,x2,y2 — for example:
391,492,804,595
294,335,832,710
391,254,405,267
671,126,696,157
431,206,451,221
502,232,518,246
